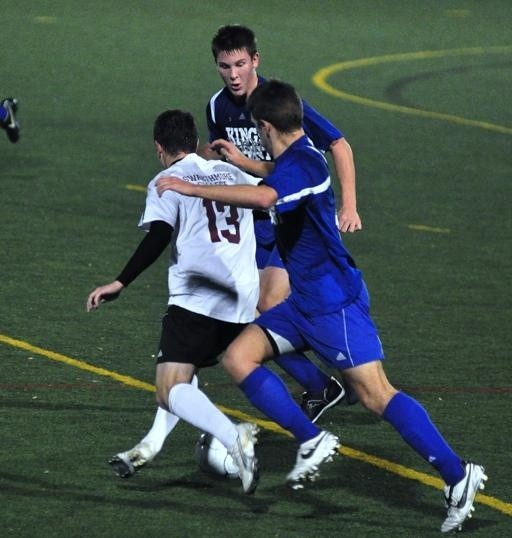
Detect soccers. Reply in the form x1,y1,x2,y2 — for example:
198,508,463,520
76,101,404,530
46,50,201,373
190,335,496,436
196,432,240,480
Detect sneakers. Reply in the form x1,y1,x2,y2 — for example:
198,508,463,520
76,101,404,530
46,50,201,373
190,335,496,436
440,460,487,534
230,423,261,496
287,430,340,491
108,450,145,480
1,98,20,143
299,375,346,426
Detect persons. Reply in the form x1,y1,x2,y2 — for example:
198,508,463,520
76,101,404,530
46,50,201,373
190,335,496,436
85,110,267,497
205,23,362,424
154,79,488,535
0,96,21,143
